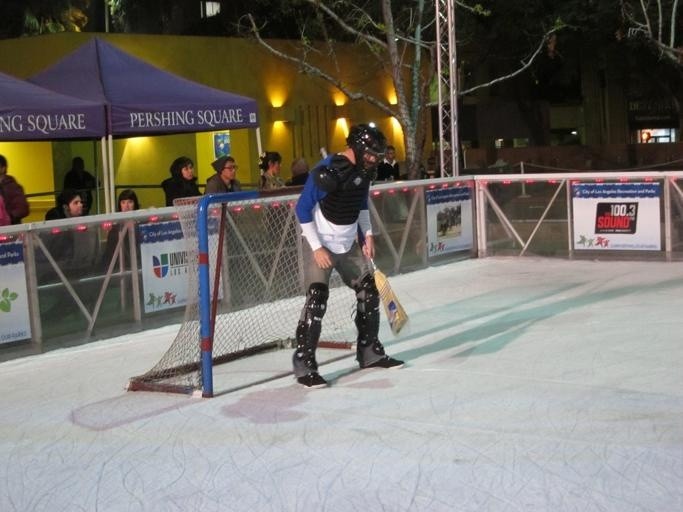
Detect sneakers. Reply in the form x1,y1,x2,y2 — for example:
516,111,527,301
296,371,327,390
359,355,405,369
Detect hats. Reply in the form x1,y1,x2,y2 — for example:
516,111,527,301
211,156,230,174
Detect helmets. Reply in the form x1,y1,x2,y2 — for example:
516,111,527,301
347,124,388,181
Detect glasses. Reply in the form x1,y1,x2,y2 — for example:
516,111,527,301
222,165,238,170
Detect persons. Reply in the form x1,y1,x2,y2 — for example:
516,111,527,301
162,157,203,207
37,155,144,320
291,122,405,389
257,151,309,246
205,155,242,194
372,146,400,181
1,156,30,242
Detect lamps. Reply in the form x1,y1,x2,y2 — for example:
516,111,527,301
271,106,287,121
335,105,350,119
387,104,399,116
